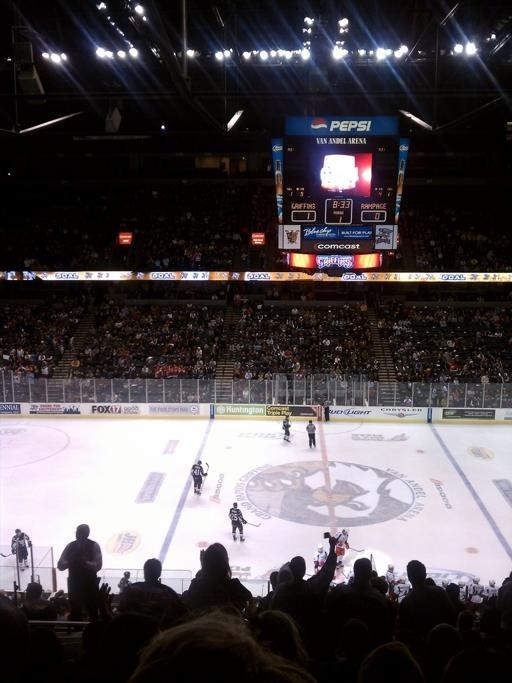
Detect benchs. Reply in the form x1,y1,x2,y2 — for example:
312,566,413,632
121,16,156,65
2,585,512,681
0,216,511,404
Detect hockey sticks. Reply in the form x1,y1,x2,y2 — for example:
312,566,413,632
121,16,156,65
201,462,209,487
247,523,262,527
0,545,32,557
349,547,365,552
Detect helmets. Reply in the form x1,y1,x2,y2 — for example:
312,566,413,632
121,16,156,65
318,543,323,551
233,502,238,507
388,564,393,569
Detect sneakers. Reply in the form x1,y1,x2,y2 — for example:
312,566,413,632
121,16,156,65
284,437,290,442
234,535,245,541
194,489,202,494
310,443,316,448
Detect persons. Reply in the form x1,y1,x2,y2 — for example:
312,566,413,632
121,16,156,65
229,503,247,542
283,415,290,442
191,460,207,495
0,525,511,682
306,420,316,448
0,207,512,422
